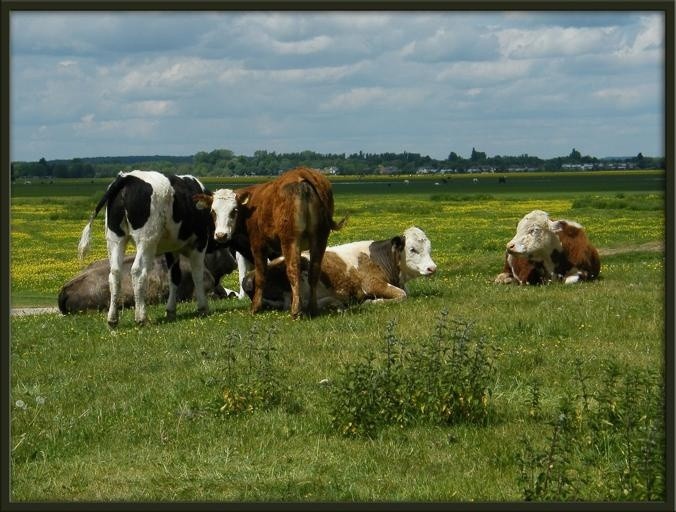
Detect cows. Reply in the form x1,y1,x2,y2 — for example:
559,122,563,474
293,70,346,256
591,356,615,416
77,169,228,327
493,209,603,285
193,168,349,315
58,242,242,317
239,226,438,311
